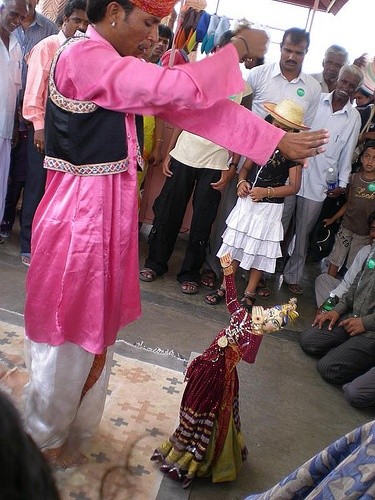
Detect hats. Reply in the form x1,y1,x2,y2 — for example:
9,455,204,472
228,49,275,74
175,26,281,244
263,99,310,131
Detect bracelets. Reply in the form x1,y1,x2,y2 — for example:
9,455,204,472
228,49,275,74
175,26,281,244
153,138,165,143
230,35,249,59
266,186,275,200
236,179,246,188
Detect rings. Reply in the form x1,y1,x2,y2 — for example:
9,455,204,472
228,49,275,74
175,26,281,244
36,143,40,147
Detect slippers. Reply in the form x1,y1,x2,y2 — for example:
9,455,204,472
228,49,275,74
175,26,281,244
180,281,200,294
139,267,157,283
200,270,216,289
21,253,31,266
288,284,305,295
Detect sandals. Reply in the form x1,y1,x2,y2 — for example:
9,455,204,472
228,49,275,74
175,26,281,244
257,284,272,299
239,294,257,311
205,286,227,305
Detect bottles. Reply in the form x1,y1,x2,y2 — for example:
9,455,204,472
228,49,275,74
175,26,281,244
322,293,335,312
326,168,337,191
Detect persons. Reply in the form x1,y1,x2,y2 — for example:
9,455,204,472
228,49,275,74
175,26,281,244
228,61,367,295
323,144,375,279
149,253,300,490
205,99,313,317
0,0,375,409
24,1,330,469
